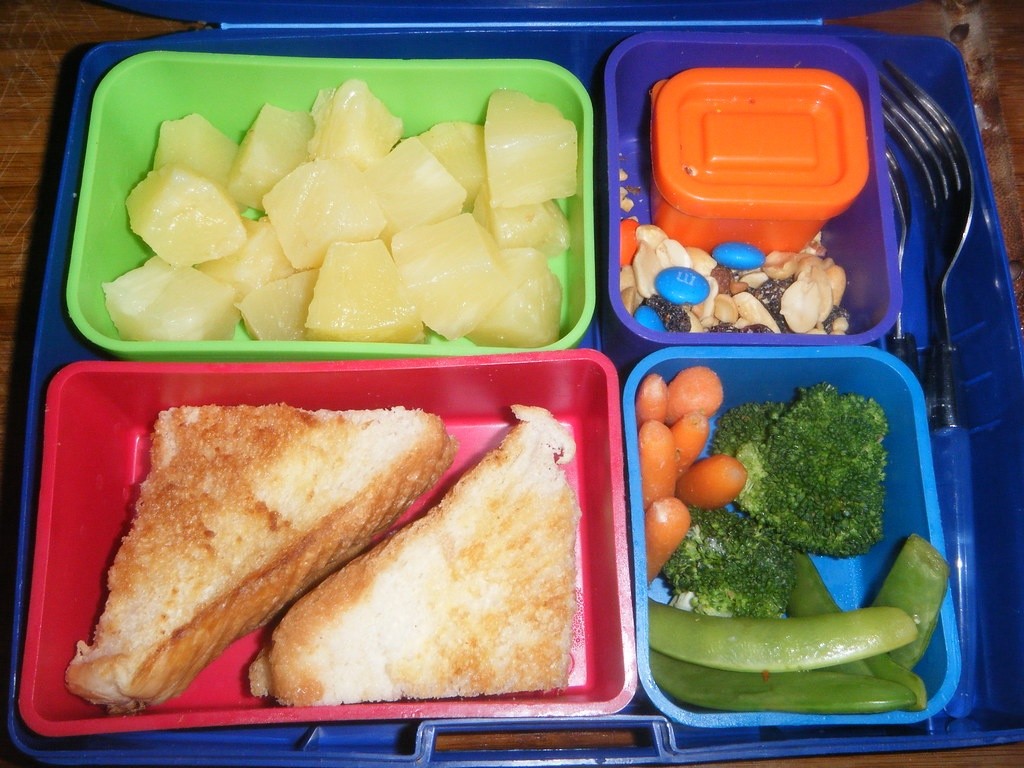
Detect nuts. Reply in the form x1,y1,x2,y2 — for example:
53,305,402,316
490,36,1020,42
616,169,849,338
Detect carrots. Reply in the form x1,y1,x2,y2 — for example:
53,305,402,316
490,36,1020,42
636,367,748,585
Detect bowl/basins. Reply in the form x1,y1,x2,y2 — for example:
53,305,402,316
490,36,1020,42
601,27,904,346
60,49,595,358
622,345,963,728
18,365,640,738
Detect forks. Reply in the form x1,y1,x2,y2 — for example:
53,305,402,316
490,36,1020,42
873,54,979,432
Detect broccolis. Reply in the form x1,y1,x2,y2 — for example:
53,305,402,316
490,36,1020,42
659,381,889,620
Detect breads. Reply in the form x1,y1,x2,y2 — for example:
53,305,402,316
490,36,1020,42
248,405,583,709
67,402,464,713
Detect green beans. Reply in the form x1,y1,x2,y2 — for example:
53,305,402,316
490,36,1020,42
644,534,951,714
